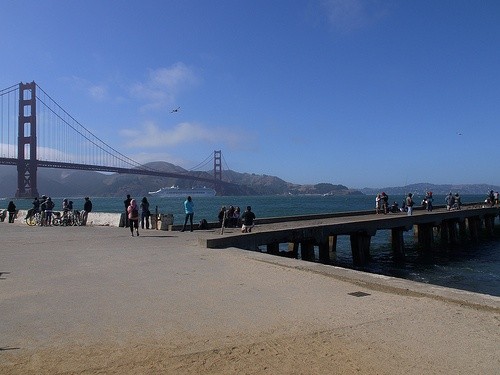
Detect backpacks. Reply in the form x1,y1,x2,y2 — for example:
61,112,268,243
130,206,138,218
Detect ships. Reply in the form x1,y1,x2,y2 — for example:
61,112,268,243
148,184,216,198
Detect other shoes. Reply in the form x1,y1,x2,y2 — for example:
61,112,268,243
131,235,133,236
136,231,139,236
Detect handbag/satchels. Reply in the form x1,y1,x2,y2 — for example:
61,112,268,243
145,210,150,216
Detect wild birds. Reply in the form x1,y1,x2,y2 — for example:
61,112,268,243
169,107,181,114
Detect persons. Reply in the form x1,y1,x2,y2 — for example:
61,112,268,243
139,197,150,229
7,201,17,223
218,205,242,228
127,198,140,237
375,190,500,216
241,206,256,233
180,196,195,232
24,194,92,227
123,194,132,228
